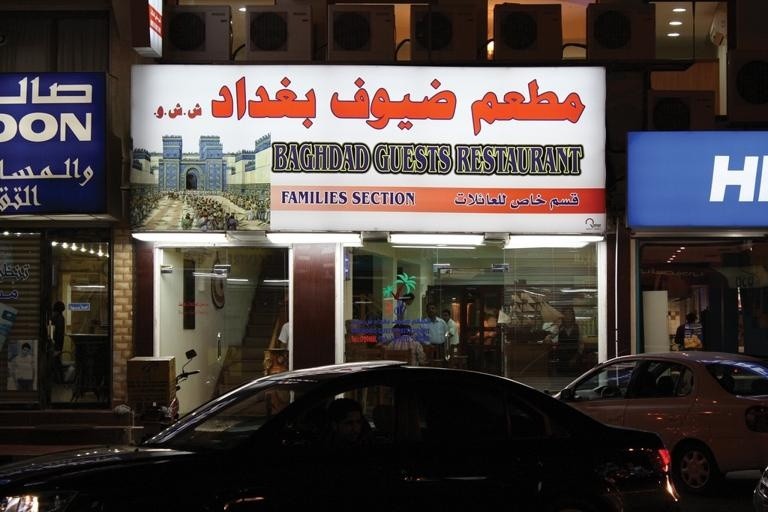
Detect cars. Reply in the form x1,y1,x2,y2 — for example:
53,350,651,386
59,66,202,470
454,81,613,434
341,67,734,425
542,351,767,495
0,359,683,511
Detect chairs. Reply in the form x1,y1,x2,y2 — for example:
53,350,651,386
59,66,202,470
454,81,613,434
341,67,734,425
385,347,440,365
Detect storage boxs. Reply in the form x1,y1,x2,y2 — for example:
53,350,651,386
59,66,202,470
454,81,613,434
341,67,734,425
125,356,176,405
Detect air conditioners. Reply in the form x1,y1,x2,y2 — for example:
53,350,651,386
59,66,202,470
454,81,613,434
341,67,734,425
161,3,232,63
326,4,399,62
586,2,657,58
244,4,313,65
492,2,563,62
409,2,488,65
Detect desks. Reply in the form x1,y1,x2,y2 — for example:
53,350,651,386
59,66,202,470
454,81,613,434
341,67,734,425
67,333,112,403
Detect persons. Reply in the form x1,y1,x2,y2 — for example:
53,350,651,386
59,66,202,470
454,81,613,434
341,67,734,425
330,397,363,448
52,300,64,385
364,294,460,366
698,311,720,351
675,313,697,350
551,349,578,381
544,306,585,356
10,344,35,391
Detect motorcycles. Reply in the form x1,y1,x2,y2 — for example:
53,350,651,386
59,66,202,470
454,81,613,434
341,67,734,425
115,348,203,446
